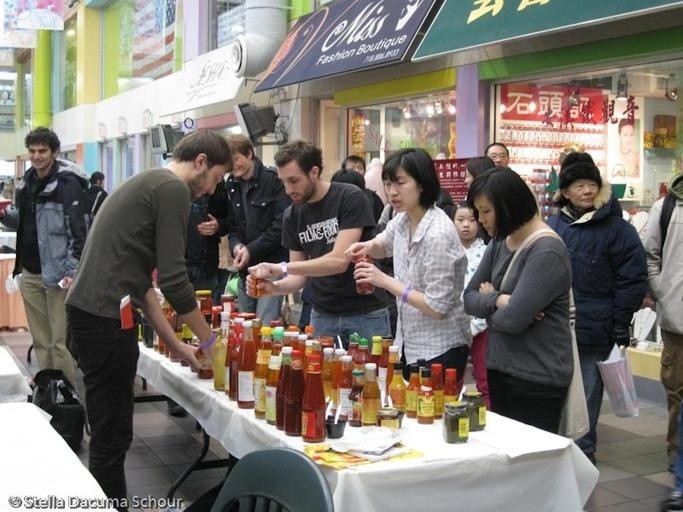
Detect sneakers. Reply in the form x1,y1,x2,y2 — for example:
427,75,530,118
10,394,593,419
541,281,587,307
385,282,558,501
660,496,682,512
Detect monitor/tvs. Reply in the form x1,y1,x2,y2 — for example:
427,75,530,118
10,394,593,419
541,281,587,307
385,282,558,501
232,101,277,141
149,124,185,153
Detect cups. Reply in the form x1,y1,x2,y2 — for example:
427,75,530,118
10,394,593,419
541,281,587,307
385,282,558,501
596,357,638,417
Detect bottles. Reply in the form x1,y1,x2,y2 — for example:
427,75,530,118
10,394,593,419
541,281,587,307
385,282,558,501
230,318,244,400
254,319,261,346
237,321,256,408
346,332,360,360
362,363,382,425
198,313,213,379
181,323,193,366
431,363,443,418
298,335,308,350
378,335,394,378
164,341,169,358
214,312,230,391
388,361,407,414
276,347,293,431
331,349,345,404
354,338,371,368
405,364,420,418
253,327,272,419
348,369,365,426
304,340,314,356
312,341,324,361
386,346,401,396
305,325,315,340
337,355,353,421
169,311,183,362
159,315,168,355
283,332,290,347
443,368,459,414
288,325,297,331
290,331,299,350
265,329,284,425
284,351,305,436
371,336,382,363
225,319,234,394
322,348,334,397
416,369,434,424
301,355,325,443
419,366,426,385
212,306,223,328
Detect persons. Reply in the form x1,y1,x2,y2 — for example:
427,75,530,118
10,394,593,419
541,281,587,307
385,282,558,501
0,127,575,511
545,149,645,462
639,174,682,473
613,119,640,176
660,404,683,512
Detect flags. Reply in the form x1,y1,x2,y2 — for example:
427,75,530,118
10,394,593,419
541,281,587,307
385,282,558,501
131,1,175,79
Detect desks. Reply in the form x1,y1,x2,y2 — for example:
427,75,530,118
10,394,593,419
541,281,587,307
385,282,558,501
0,345,33,403
0,402,119,512
134,338,600,512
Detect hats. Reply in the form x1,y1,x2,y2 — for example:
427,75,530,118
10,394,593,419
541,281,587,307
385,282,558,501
559,153,602,189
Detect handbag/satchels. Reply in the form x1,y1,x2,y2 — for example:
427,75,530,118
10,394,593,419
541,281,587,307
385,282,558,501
560,320,591,440
28,368,90,452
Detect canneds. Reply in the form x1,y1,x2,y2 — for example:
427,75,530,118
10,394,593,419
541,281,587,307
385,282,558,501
212,306,221,325
443,400,469,444
354,254,375,295
376,408,400,428
460,391,486,431
197,344,214,379
195,290,212,314
222,294,234,311
249,276,265,297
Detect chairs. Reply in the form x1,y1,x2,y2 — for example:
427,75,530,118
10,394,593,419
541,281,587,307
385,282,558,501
209,447,334,512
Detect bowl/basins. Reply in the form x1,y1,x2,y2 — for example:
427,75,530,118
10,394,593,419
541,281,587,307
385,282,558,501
326,419,346,438
